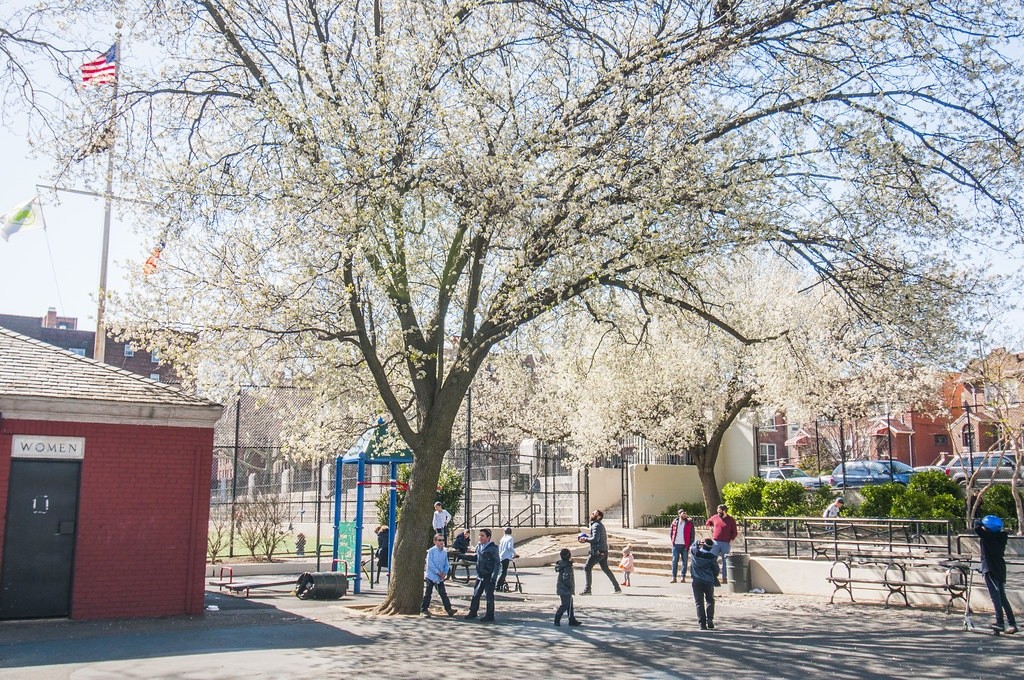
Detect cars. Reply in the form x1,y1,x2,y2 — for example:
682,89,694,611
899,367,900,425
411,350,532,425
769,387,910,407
830,460,915,488
759,468,828,489
912,466,945,473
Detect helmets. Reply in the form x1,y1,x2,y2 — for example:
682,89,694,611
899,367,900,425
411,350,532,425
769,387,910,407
981,515,1003,533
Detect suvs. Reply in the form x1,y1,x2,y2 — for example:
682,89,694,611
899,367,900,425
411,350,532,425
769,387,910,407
944,452,1024,487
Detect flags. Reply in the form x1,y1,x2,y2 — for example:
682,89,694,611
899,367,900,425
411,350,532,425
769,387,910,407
79,43,116,87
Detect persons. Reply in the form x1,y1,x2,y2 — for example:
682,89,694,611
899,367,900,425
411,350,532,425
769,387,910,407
670,509,695,583
420,533,458,618
554,548,582,626
453,529,471,573
432,502,451,547
578,510,621,595
706,504,738,584
496,527,519,591
975,515,1018,634
374,525,389,583
690,538,720,629
823,498,844,529
295,532,306,555
619,543,635,586
458,529,500,621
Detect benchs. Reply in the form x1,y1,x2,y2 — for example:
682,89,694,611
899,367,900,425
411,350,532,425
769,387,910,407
803,521,972,612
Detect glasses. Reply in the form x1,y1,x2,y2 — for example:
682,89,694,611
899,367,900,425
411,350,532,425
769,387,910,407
436,539,445,541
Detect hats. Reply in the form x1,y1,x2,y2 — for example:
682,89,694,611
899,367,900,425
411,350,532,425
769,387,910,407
703,538,713,551
623,544,632,554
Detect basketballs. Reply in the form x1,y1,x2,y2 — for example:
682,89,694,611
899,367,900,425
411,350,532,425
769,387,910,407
578,533,589,543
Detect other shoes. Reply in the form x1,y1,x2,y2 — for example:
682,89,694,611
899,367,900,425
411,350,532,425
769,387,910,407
1003,625,1018,634
373,580,379,584
680,579,686,583
612,589,622,594
700,626,707,630
719,579,727,583
991,622,1004,631
420,611,431,618
450,610,457,616
461,561,470,566
708,620,714,628
554,620,560,626
481,614,494,622
671,578,677,583
627,584,630,587
569,621,582,626
579,590,592,596
452,575,457,580
465,610,477,619
621,583,626,586
